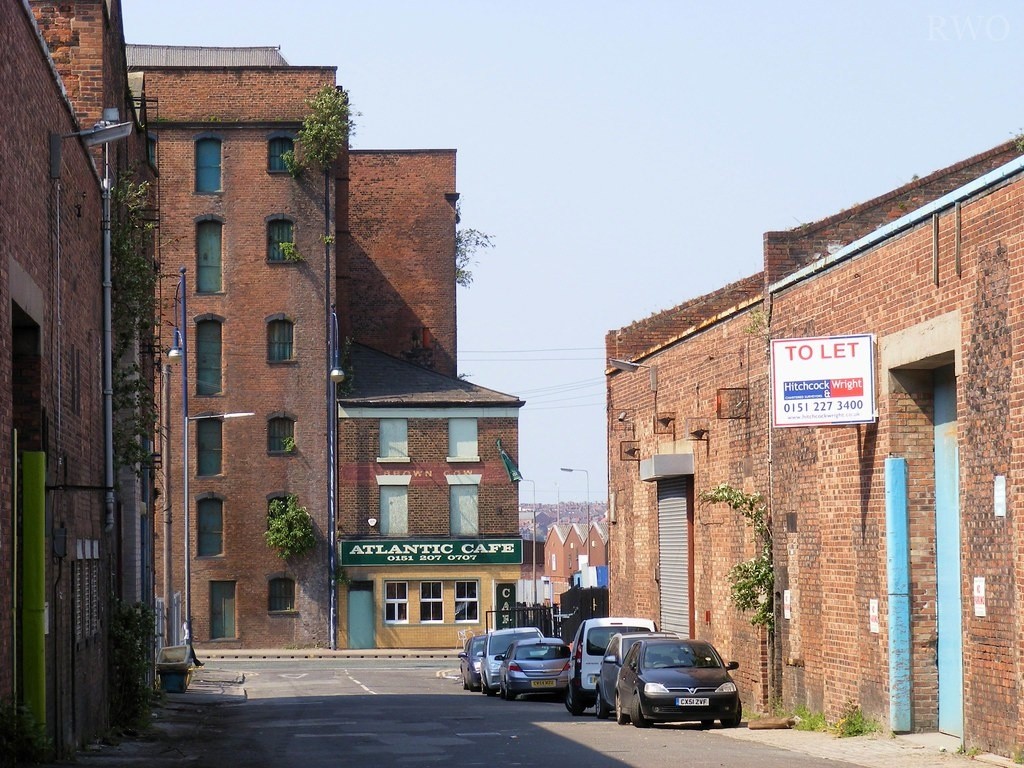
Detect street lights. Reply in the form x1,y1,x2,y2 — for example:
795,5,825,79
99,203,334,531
561,468,590,567
168,266,203,668
329,304,344,651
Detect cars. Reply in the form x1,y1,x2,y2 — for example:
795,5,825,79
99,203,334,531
614,638,741,728
595,631,691,719
476,627,549,696
495,637,571,700
457,635,487,692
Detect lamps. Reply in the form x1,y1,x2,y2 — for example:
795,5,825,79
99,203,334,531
657,417,675,427
624,448,640,458
50,121,134,178
690,428,708,439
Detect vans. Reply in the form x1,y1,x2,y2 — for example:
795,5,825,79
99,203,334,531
566,616,657,715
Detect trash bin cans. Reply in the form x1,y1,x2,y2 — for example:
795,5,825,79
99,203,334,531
156,644,195,693
